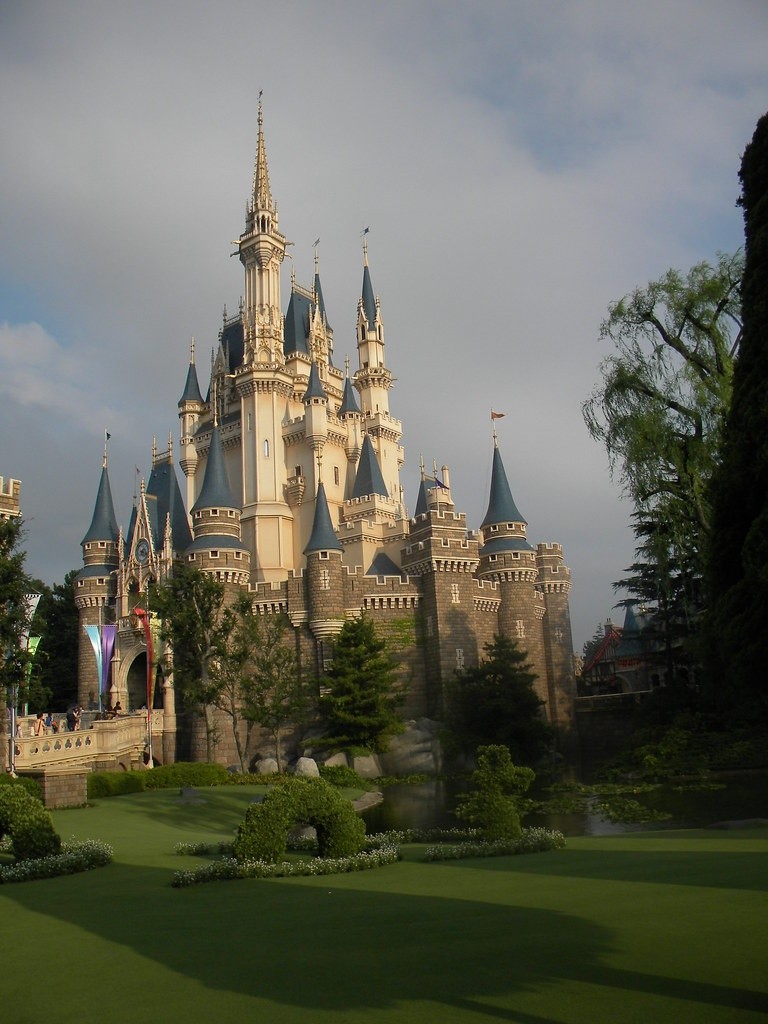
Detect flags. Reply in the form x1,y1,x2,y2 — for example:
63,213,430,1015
435,479,449,490
491,412,505,419
106,432,111,440
136,468,141,474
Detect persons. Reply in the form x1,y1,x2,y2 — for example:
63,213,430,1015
16,701,147,753
